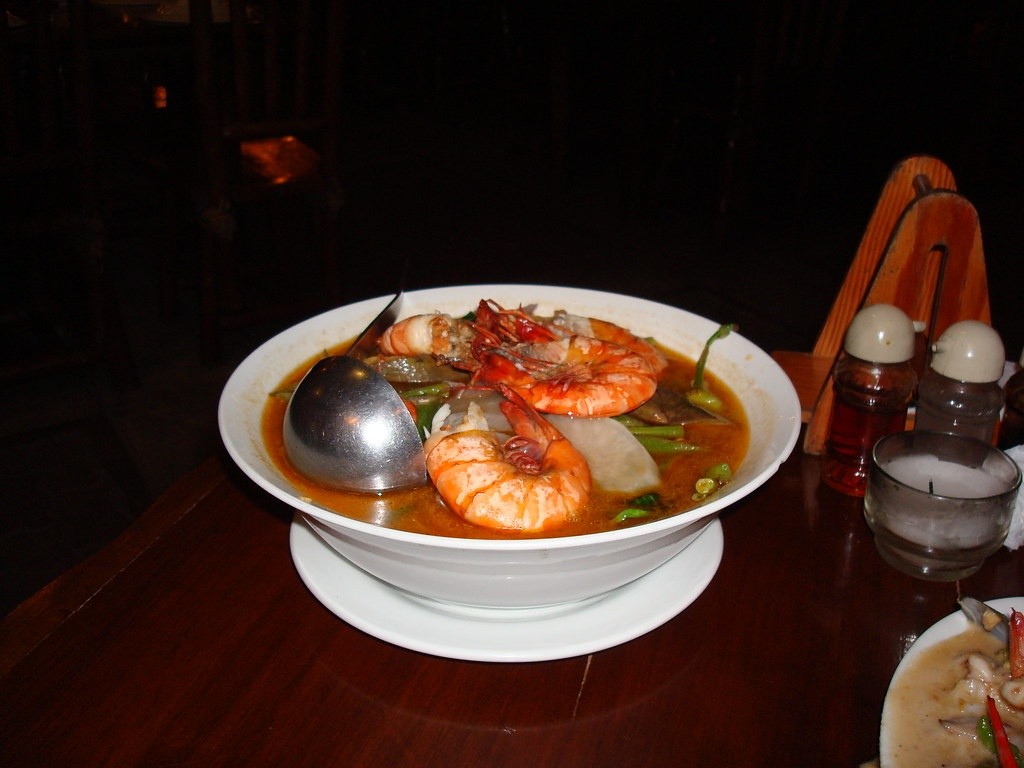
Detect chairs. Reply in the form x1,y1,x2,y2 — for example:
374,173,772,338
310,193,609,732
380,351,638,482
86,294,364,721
0,0,350,389
644,0,849,246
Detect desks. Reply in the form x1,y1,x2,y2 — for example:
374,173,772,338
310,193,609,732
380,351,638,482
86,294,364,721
0,417,1024,768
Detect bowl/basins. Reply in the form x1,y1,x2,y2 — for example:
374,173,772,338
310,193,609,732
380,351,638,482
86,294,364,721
217,284,802,608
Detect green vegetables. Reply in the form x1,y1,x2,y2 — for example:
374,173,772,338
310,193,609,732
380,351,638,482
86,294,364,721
261,310,735,526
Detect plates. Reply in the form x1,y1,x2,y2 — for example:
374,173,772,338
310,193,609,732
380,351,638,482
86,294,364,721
288,512,722,662
879,597,1024,767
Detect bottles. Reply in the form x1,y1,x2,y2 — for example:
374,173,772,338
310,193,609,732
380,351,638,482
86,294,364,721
998,345,1024,450
919,322,1018,452
813,305,928,515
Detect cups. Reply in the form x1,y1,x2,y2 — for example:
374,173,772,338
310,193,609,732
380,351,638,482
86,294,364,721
861,431,1021,583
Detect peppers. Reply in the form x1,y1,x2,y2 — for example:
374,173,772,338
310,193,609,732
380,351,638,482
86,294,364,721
978,695,1024,768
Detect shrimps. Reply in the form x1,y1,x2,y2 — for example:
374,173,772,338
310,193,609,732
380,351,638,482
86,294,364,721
377,297,669,532
